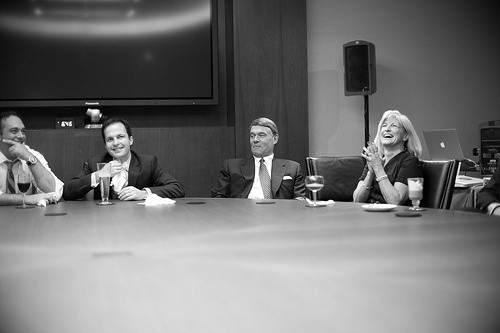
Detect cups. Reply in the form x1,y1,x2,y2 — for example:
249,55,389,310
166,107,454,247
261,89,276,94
96,162,113,205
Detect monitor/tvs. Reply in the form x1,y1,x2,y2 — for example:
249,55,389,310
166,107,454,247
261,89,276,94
0,0,219,106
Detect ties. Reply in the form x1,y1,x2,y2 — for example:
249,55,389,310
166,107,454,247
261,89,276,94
3,161,19,194
259,158,273,200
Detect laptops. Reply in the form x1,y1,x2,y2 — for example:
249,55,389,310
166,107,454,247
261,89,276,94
423,128,479,173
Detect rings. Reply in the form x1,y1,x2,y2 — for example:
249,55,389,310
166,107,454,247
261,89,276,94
368,153,371,156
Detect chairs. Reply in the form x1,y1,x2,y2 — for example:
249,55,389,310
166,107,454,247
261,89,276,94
307,156,365,202
418,158,458,210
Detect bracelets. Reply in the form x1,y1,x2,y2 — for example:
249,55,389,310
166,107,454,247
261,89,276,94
376,175,388,182
489,204,500,215
361,181,372,190
21,193,26,206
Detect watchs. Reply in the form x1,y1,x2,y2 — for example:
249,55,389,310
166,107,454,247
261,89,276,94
26,154,38,166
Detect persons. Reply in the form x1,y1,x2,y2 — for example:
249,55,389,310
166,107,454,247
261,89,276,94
353,109,425,206
476,158,500,216
62,117,186,201
0,110,64,205
210,117,306,200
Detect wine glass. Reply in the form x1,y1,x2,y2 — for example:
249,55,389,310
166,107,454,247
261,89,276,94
17,174,31,207
407,178,426,211
305,175,325,207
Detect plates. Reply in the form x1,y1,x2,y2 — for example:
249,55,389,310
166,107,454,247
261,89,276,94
361,202,397,212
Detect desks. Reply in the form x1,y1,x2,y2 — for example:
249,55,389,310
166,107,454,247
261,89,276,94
0,197,500,333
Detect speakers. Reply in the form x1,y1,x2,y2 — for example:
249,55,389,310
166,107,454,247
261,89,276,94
342,40,376,97
